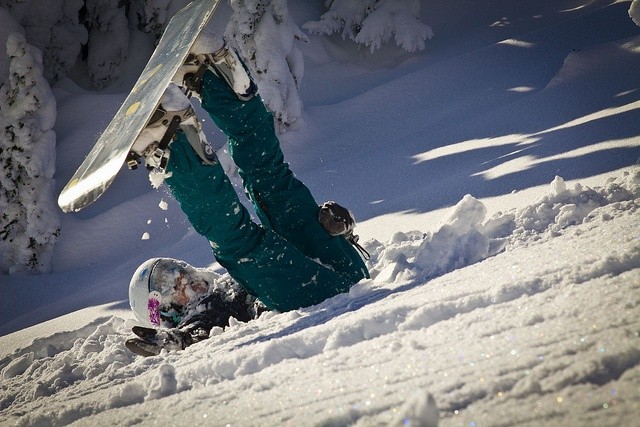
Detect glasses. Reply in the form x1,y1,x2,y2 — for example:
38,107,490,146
149,258,191,297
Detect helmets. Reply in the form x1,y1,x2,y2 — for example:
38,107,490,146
128,257,202,330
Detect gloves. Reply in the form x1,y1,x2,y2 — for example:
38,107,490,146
125,326,193,355
316,201,354,235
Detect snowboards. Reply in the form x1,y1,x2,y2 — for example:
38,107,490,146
57,0,220,212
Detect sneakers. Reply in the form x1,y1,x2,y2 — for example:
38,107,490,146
124,82,195,171
172,28,225,100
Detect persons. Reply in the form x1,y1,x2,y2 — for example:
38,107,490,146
125,37,367,353
